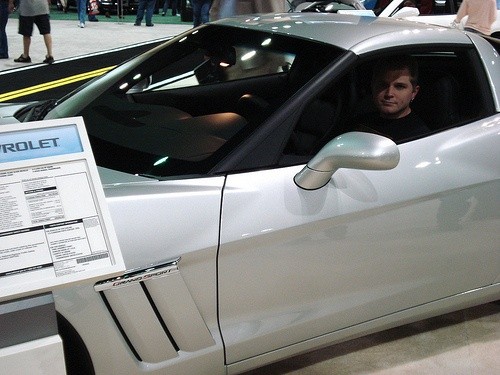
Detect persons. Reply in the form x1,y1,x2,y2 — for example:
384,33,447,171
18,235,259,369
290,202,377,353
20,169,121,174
338,50,432,145
8,0,55,65
189,0,212,29
454,0,498,37
134,0,156,27
76,0,86,28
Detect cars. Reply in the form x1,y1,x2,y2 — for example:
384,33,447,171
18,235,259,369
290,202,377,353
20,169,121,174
0,13,500,374
300,0,500,54
56,0,139,15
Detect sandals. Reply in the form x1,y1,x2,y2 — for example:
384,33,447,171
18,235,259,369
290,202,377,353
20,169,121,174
14,54,30,62
43,55,55,63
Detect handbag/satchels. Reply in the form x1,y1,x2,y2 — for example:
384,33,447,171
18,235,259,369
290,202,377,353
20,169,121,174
88,0,99,15
